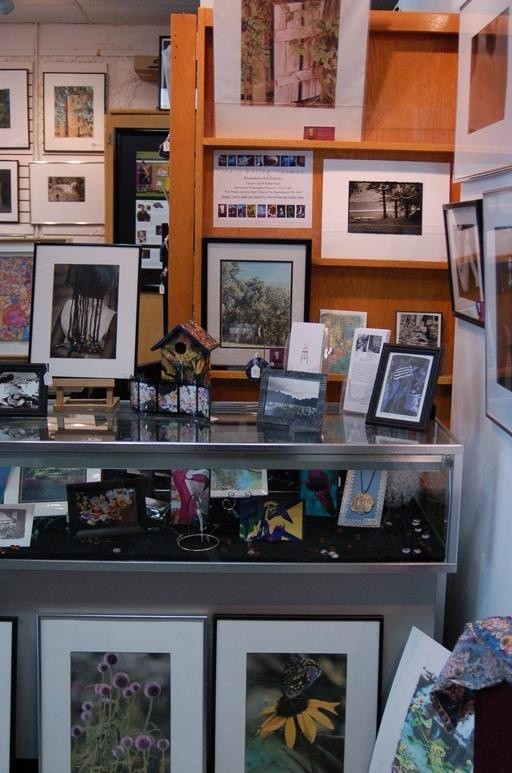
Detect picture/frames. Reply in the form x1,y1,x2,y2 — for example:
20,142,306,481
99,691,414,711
35,612,210,773
364,342,442,433
0,68,31,150
0,615,19,773
209,467,269,499
481,186,512,438
0,159,20,224
28,160,106,226
207,612,383,773
442,198,486,328
337,470,389,528
66,477,149,541
256,368,329,434
26,241,144,380
42,71,107,153
3,464,103,517
0,502,35,548
0,361,49,419
201,236,311,370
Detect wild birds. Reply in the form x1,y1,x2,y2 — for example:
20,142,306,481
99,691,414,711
172,465,211,524
251,500,293,542
